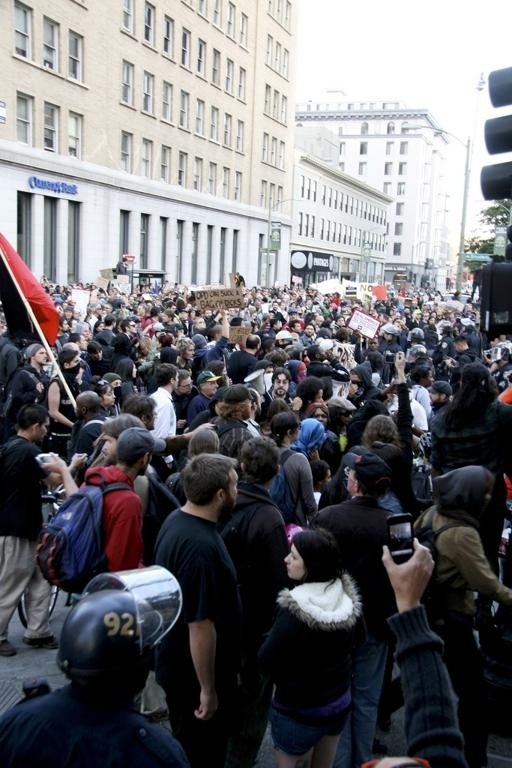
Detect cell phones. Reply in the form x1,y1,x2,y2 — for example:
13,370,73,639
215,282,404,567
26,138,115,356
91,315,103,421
384,510,416,565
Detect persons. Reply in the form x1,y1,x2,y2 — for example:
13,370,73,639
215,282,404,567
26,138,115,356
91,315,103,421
1,260,512,768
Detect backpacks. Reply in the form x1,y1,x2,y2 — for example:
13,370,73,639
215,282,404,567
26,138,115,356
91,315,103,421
412,464,434,499
35,482,134,607
417,506,480,593
268,449,300,522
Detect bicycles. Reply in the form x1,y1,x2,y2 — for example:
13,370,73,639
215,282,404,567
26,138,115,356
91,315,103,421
18,483,72,629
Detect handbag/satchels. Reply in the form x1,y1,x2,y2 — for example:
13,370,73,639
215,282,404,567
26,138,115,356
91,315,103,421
1,394,13,417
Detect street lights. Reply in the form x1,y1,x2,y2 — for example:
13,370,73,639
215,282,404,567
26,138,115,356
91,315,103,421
263,196,304,287
401,122,472,300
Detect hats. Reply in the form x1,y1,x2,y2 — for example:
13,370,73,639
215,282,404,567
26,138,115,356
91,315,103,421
327,396,357,410
103,373,122,384
26,343,42,363
116,427,167,460
196,370,222,386
342,452,393,495
222,384,254,405
427,380,453,398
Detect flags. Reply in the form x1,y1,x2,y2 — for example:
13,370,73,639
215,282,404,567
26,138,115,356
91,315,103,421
0,233,60,346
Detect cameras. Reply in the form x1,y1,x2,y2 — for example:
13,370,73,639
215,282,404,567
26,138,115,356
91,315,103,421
383,352,401,364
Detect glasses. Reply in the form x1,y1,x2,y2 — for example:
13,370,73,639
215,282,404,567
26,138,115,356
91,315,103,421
178,379,194,389
342,410,353,417
297,422,302,430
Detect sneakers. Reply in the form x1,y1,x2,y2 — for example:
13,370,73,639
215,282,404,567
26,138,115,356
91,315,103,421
1,639,17,657
22,635,58,650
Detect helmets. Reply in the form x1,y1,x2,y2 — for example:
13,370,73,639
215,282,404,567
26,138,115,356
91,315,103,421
319,339,336,354
275,331,293,341
493,341,512,364
460,317,475,334
436,320,454,336
54,563,183,698
407,328,425,344
383,323,401,339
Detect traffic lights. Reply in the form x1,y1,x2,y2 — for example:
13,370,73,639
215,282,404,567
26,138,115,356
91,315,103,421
476,252,512,340
477,65,512,202
